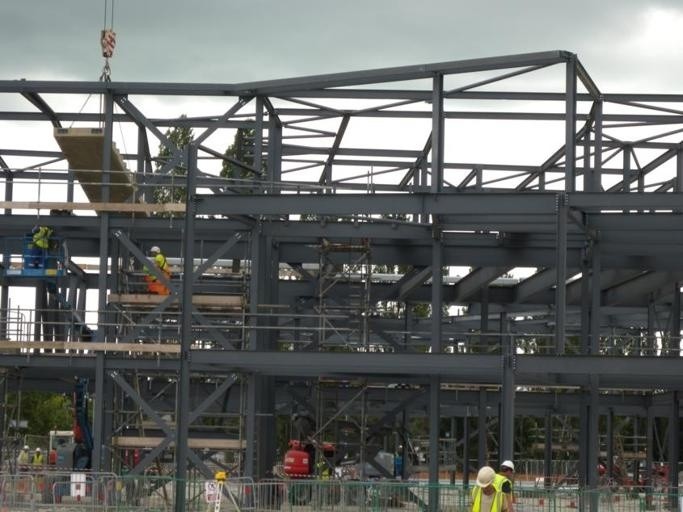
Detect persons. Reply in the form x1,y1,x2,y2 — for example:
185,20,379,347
17,445,57,493
471,460,514,512
27,224,54,267
143,246,172,296
316,456,331,506
395,445,403,476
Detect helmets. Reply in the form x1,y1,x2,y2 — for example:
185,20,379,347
476,466,496,488
36,448,41,452
501,460,514,472
23,445,30,449
151,246,160,254
